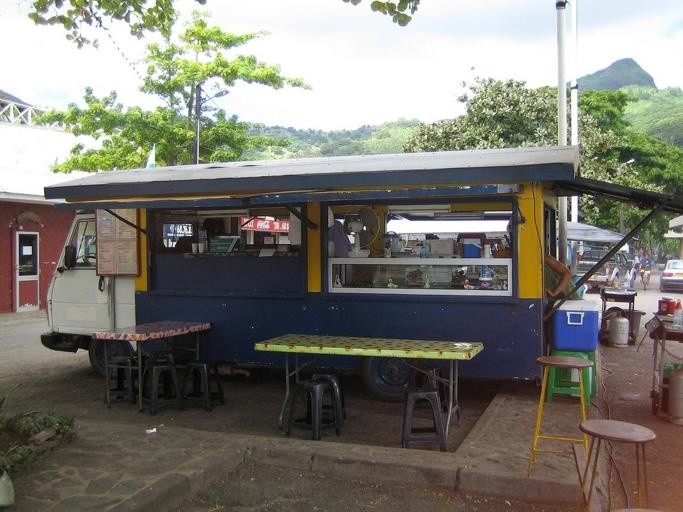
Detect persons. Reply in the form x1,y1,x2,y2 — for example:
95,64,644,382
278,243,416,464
544,255,579,299
624,247,658,290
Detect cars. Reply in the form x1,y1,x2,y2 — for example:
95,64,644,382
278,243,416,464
658,260,681,293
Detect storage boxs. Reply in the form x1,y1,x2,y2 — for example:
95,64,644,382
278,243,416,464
551,299,599,351
458,237,481,258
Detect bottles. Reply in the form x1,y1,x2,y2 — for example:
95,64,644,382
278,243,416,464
420,247,429,258
383,241,392,257
673,299,683,330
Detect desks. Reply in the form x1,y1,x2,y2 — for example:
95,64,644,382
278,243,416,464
601,286,636,338
651,316,683,418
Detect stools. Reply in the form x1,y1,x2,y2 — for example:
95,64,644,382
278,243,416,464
407,366,441,391
313,368,346,426
185,361,225,411
526,354,593,481
286,377,339,443
577,419,656,512
103,354,138,405
546,349,596,404
400,385,446,450
141,357,185,414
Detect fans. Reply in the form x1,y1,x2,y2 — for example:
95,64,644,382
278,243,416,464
340,206,379,258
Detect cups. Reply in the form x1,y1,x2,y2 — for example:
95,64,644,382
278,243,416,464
484,245,490,258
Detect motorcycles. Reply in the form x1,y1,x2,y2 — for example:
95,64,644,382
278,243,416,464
638,264,652,291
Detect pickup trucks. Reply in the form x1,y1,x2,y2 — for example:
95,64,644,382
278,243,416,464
568,249,639,296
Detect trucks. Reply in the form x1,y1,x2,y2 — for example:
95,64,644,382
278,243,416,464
35,143,583,404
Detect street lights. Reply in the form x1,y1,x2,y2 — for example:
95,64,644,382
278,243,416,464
191,86,230,164
618,153,638,237
